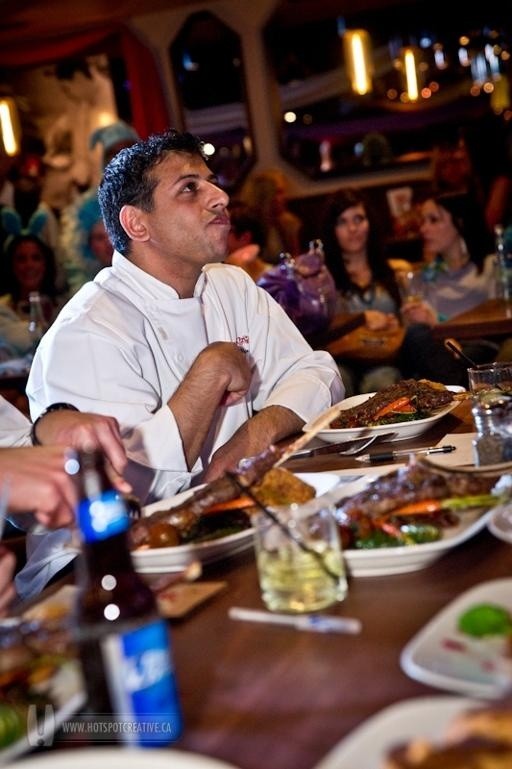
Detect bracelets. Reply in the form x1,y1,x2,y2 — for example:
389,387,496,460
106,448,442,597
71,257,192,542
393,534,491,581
26,403,79,446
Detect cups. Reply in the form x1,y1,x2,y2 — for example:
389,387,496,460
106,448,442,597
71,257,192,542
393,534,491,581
250,504,351,614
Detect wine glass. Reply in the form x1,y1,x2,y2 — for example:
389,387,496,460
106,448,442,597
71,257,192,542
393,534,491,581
395,270,426,323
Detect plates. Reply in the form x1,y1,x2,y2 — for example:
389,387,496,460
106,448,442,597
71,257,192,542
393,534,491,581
5,749,243,769
1,614,90,766
396,582,511,703
126,362,510,575
313,698,511,768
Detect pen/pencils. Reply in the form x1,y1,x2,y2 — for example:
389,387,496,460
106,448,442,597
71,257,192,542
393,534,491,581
227,606,363,635
355,445,456,465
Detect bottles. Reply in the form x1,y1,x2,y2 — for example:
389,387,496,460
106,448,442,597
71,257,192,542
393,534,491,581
69,445,186,746
492,225,512,305
25,291,48,369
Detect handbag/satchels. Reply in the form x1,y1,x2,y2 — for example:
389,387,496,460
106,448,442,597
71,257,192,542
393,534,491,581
256,251,337,344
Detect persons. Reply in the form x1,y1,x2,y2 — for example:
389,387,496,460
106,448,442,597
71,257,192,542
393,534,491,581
1,110,510,395
23,129,346,505
1,392,131,560
